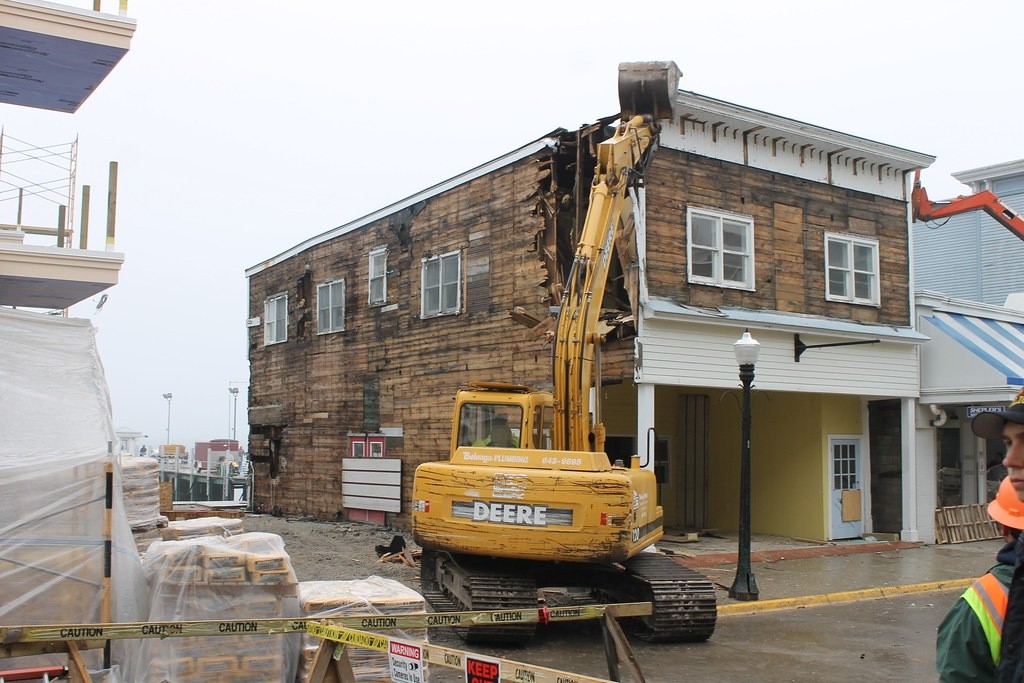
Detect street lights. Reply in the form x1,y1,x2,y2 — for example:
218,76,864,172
728,328,759,601
163,393,172,444
228,387,239,440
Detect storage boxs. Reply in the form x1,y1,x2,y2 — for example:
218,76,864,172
298,579,431,683
144,533,299,683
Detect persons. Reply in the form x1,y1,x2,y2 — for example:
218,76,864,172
472,412,519,448
972,388,1024,683
987,452,1004,469
936,475,1024,683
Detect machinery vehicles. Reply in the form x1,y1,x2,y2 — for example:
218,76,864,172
414,58,718,647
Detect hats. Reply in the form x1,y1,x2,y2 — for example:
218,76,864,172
970,385,1024,439
492,412,508,425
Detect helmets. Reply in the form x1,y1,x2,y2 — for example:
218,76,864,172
986,475,1024,529
